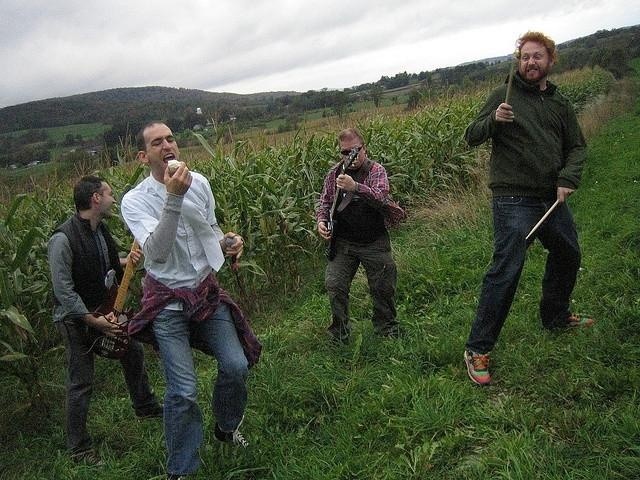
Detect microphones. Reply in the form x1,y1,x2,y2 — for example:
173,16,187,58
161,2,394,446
226,238,237,274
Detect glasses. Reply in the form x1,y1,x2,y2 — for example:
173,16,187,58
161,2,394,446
340,144,364,156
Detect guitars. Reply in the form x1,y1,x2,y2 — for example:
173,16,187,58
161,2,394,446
326,143,362,260
89,236,140,358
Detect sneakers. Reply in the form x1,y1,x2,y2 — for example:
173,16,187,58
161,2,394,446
213,414,250,450
463,349,492,386
133,402,165,421
70,448,105,467
559,316,594,328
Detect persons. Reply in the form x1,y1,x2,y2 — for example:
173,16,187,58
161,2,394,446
315,127,409,345
48,175,165,466
121,120,263,480
462,31,596,384
